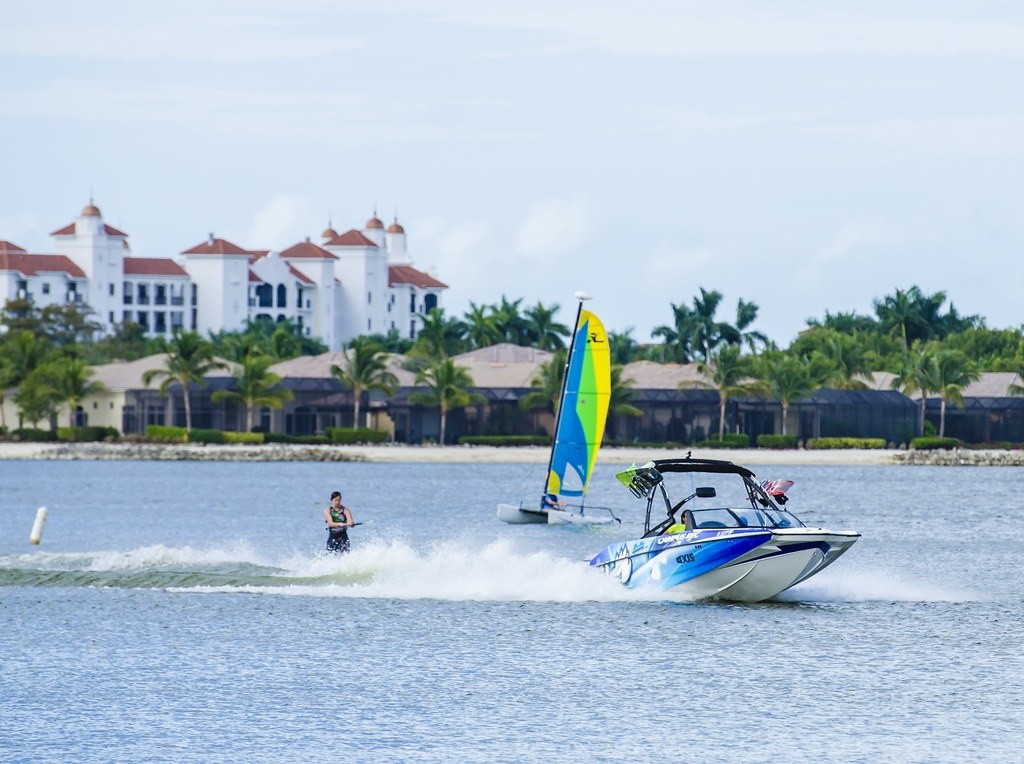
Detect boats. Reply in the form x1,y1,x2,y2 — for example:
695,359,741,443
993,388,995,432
585,451,862,608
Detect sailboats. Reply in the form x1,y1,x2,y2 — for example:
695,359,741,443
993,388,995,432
497,293,622,528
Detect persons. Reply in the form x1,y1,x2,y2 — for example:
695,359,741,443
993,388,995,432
325,490,354,560
662,509,694,536
